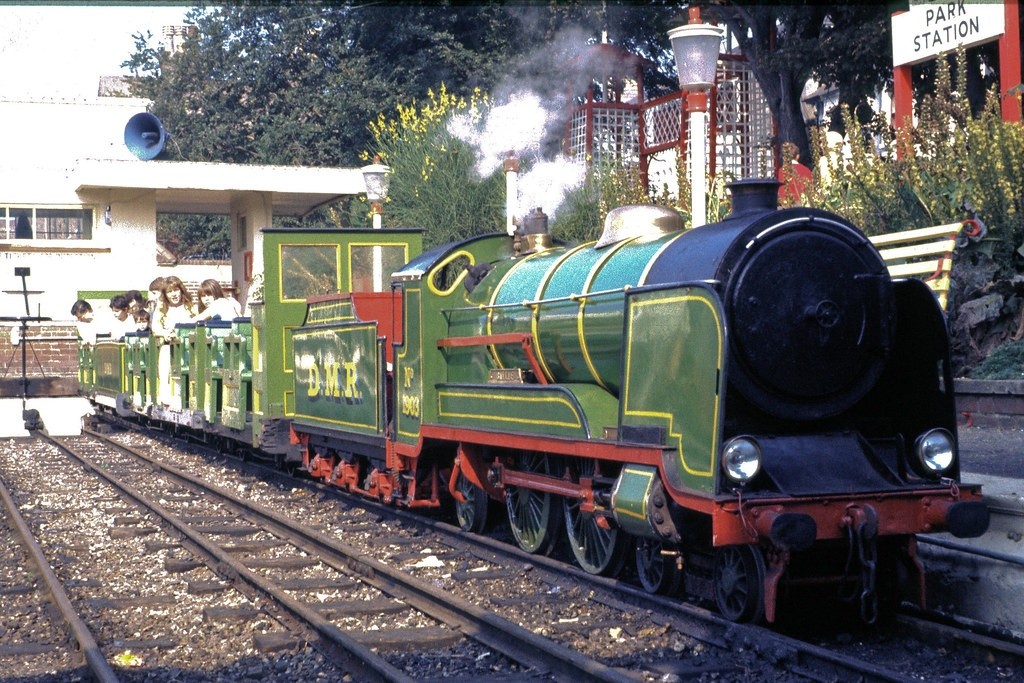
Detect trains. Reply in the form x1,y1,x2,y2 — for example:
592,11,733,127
73,220,992,624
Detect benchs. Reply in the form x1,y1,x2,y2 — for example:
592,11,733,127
867,218,980,312
75,316,253,430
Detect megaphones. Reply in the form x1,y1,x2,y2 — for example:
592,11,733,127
123,112,175,161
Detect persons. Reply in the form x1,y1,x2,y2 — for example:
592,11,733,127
778,142,813,203
70,276,242,347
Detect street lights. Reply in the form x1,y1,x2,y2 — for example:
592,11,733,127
360,154,395,294
665,5,724,228
487,102,526,236
